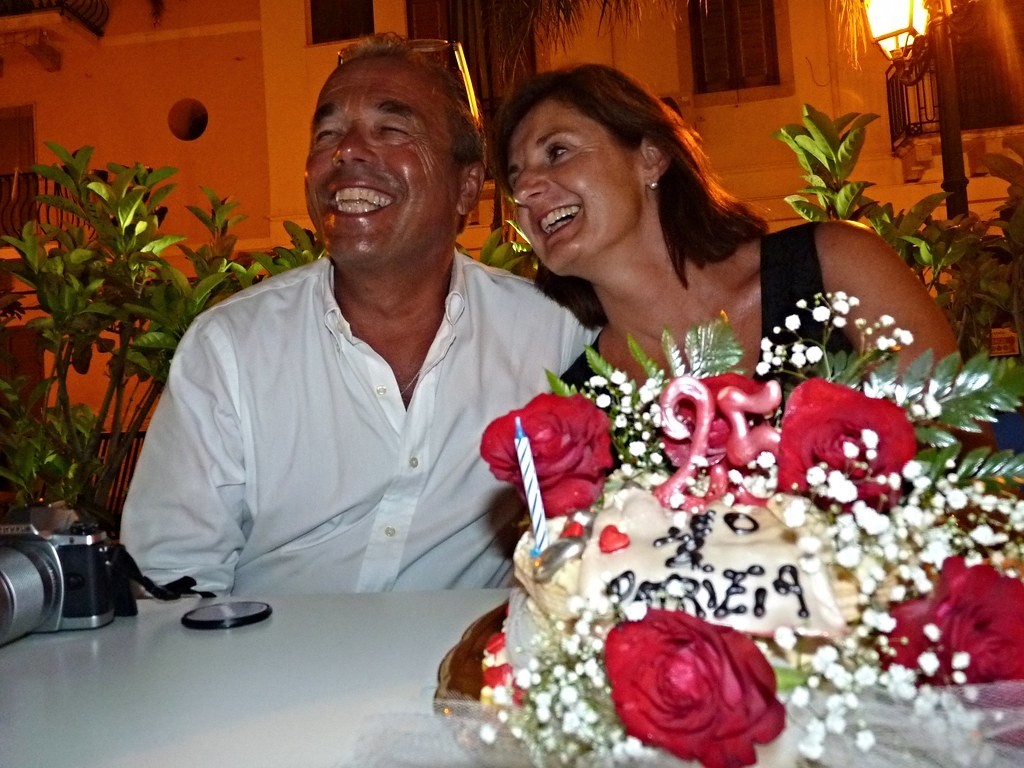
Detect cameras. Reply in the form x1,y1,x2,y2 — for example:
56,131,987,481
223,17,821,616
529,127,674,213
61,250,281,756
0,497,116,646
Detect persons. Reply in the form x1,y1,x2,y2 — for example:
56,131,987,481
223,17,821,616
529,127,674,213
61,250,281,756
486,63,998,493
119,28,601,600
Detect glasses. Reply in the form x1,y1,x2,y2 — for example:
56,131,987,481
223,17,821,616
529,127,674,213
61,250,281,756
337,36,483,128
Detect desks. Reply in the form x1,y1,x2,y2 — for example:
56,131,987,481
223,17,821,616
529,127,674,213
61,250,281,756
0,587,513,768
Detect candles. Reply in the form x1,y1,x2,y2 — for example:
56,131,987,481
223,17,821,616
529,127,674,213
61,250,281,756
659,379,728,509
514,416,548,553
717,379,783,508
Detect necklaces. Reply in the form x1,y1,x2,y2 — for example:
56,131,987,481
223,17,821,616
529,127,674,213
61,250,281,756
399,369,420,399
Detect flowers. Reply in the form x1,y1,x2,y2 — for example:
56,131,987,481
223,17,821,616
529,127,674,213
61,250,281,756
481,290,1024,768
